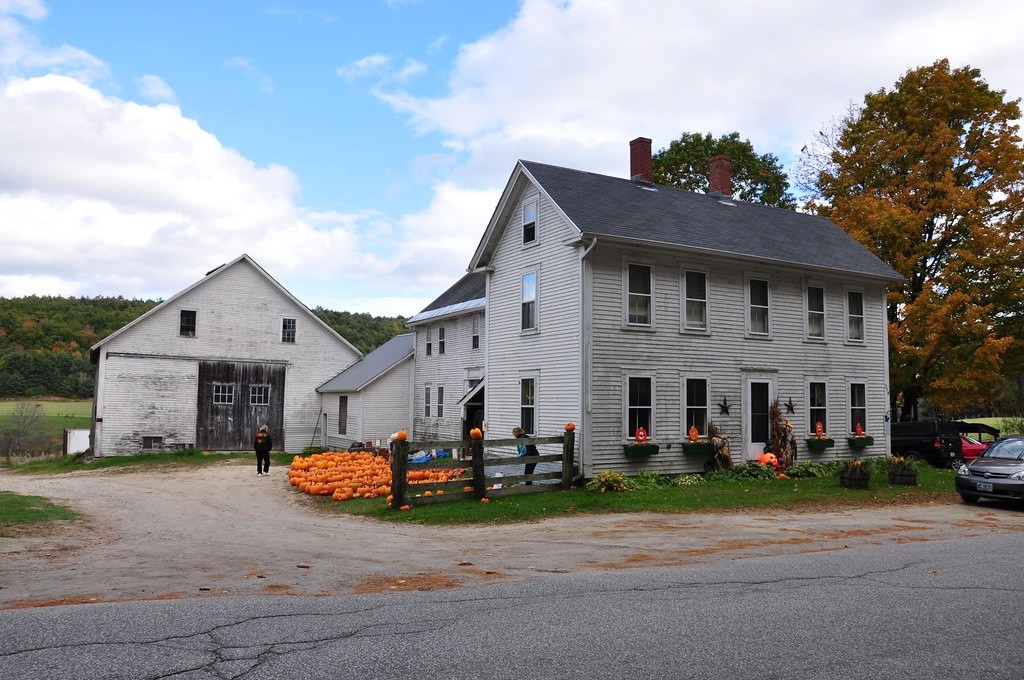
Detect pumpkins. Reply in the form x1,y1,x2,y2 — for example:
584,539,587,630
390,432,398,441
290,451,491,509
565,423,575,432
398,429,407,441
470,427,482,439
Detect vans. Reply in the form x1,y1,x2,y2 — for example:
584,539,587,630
892,420,949,466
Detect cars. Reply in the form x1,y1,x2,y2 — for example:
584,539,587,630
953,435,1024,511
937,435,992,471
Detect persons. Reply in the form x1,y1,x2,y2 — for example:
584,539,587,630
254,425,272,477
511,427,540,486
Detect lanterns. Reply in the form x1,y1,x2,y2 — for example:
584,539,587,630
759,453,777,468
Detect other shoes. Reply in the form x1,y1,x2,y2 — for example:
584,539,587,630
263,471,270,476
257,474,262,477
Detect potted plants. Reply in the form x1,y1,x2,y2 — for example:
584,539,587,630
682,440,715,456
847,435,875,448
807,437,835,449
884,453,919,486
625,441,660,457
832,459,872,490
